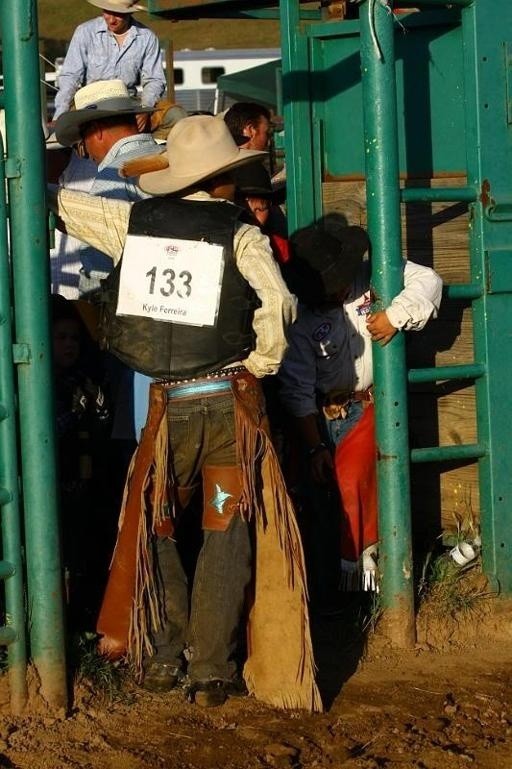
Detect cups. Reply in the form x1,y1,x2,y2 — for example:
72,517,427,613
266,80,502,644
450,542,475,566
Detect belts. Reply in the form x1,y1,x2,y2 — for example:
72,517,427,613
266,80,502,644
157,365,247,389
351,383,374,402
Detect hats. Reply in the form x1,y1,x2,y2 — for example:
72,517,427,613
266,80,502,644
86,0,149,13
55,79,161,147
138,115,271,197
237,163,286,205
288,213,370,305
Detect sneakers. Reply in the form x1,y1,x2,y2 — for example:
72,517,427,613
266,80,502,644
140,665,178,693
193,680,227,707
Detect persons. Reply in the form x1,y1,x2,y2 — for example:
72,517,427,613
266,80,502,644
52,0,188,140
45,79,443,707
